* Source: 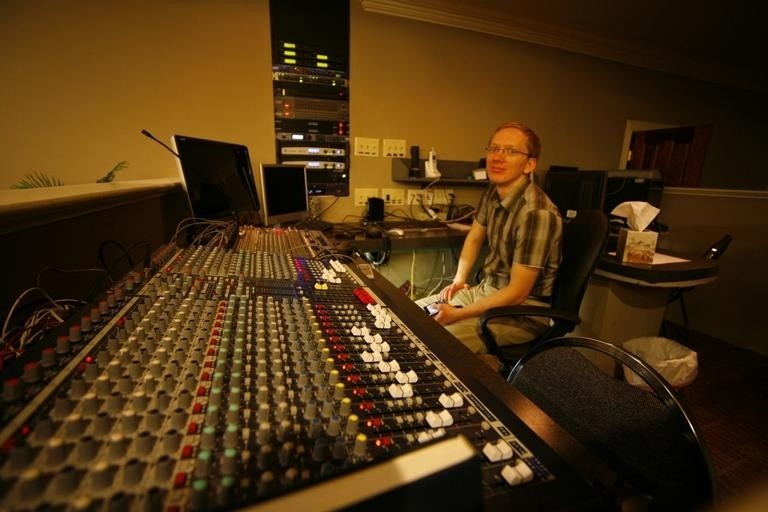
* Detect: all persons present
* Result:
[410,117,568,357]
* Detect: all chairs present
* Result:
[480,206,610,379]
[666,228,733,326]
[506,335,719,512]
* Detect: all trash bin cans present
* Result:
[622,336,699,394]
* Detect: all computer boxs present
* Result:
[604,177,664,219]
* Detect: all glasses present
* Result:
[485,145,535,158]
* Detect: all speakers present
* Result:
[366,197,384,222]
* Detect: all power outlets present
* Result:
[354,136,454,208]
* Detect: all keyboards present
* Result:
[310,221,332,231]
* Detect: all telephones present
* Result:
[425,147,442,178]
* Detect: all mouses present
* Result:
[334,230,356,239]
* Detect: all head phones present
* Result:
[363,228,391,267]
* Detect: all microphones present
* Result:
[140,128,180,158]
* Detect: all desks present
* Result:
[322,220,470,253]
[351,253,623,512]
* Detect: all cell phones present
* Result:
[423,300,444,316]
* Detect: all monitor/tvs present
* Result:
[545,169,605,218]
[259,163,310,227]
[170,133,261,222]
